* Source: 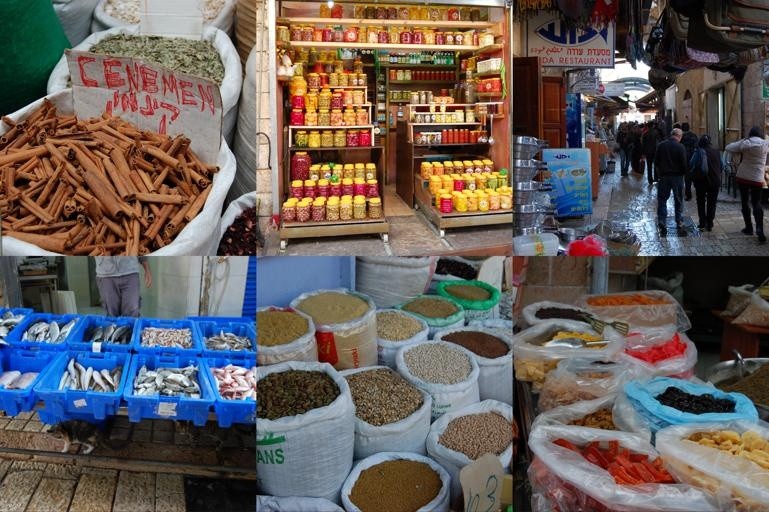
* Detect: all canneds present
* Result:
[276,1,513,224]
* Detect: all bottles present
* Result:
[472,32,478,46]
[412,70,455,81]
[397,102,404,120]
[378,49,454,65]
[442,128,468,143]
[350,48,374,58]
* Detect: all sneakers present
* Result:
[677,220,684,228]
[658,222,667,229]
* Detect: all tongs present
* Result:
[585,314,630,335]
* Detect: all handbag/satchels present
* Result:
[723,137,746,166]
[688,144,709,178]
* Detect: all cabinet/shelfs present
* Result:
[386,65,458,180]
[477,43,506,120]
[276,39,294,81]
[375,60,389,182]
[395,103,511,237]
[278,146,389,250]
[285,52,375,147]
[277,16,492,52]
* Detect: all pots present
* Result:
[558,227,587,242]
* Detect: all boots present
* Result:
[741,207,753,235]
[753,210,766,243]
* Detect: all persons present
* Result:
[726,126,767,243]
[690,134,723,229]
[655,128,689,230]
[94,256,151,316]
[616,120,698,199]
